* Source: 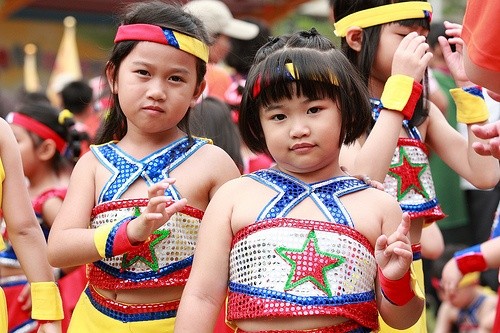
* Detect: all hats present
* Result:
[180,0,260,40]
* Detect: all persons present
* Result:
[0,76,116,333]
[46,0,241,333]
[331,0,500,333]
[181,0,259,176]
[172,26,424,333]
[0,116,65,333]
